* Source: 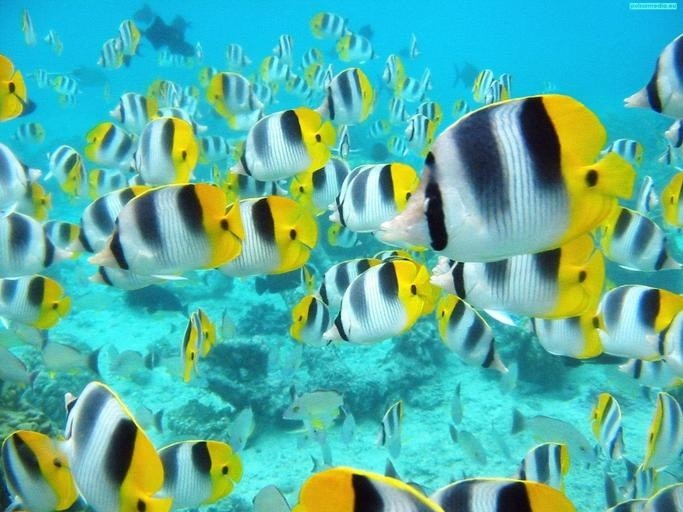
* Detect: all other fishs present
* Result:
[0,2,682,512]
